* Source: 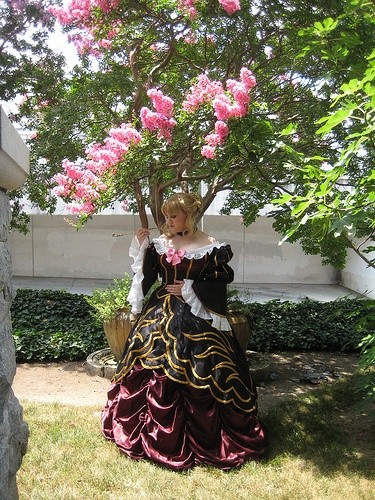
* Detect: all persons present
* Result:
[100,191,271,474]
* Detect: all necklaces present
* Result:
[175,227,196,236]
